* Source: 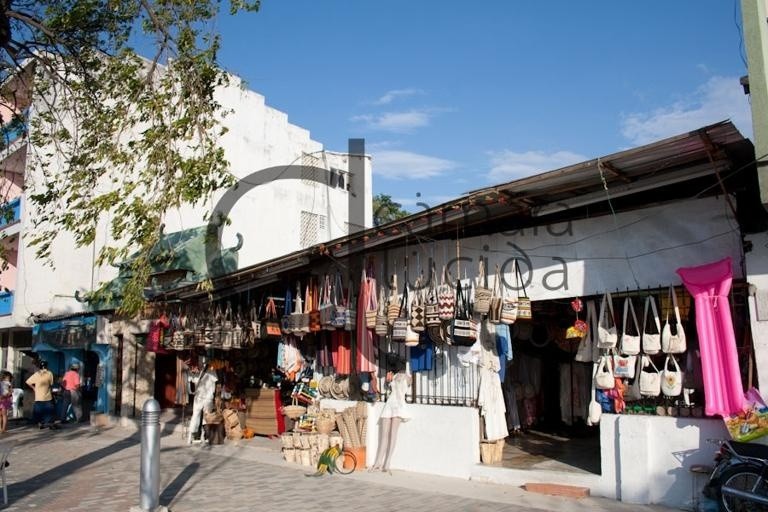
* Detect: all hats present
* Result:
[71,363,81,369]
[317,374,364,401]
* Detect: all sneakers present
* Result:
[39,423,62,431]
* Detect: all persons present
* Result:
[11,386,25,418]
[0,370,13,400]
[187,369,219,446]
[0,369,13,434]
[25,361,62,430]
[61,363,84,424]
[370,368,410,474]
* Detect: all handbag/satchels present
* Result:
[574,290,688,426]
[721,387,768,442]
[159,285,356,349]
[366,260,531,347]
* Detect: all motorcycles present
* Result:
[702,438,767,510]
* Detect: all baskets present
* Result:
[280,398,344,472]
